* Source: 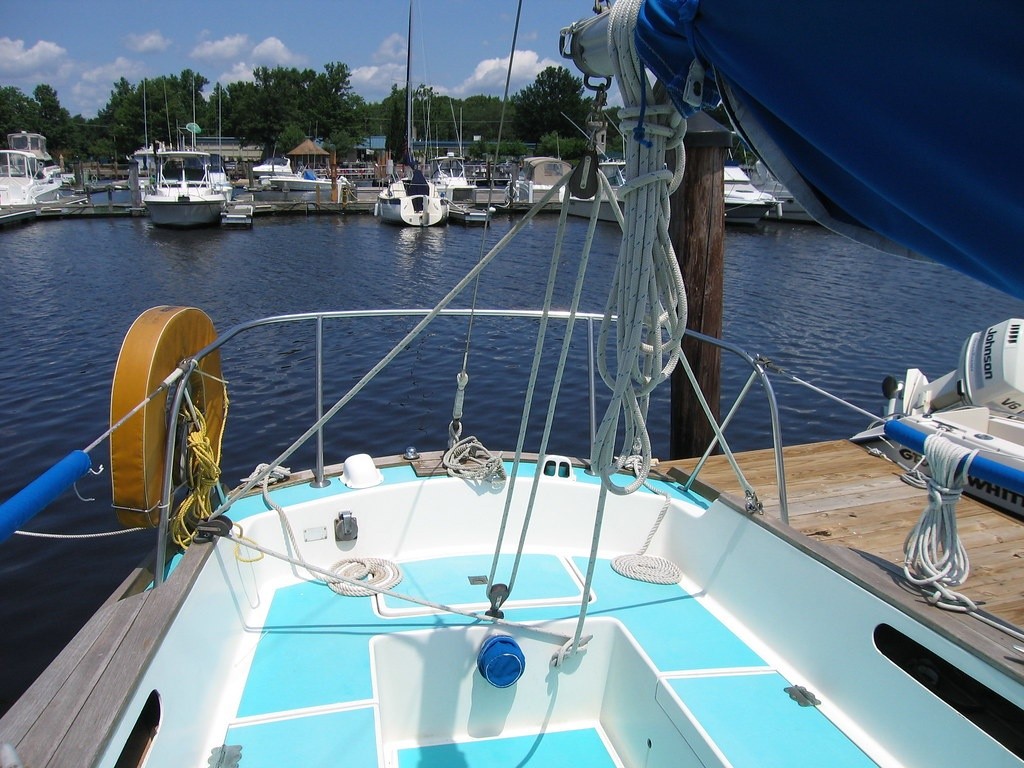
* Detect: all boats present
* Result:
[563,161,626,222]
[249,173,357,202]
[253,156,294,177]
[0,128,65,226]
[724,161,824,225]
[414,88,477,200]
[132,76,235,226]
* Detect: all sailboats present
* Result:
[375,0,446,228]
